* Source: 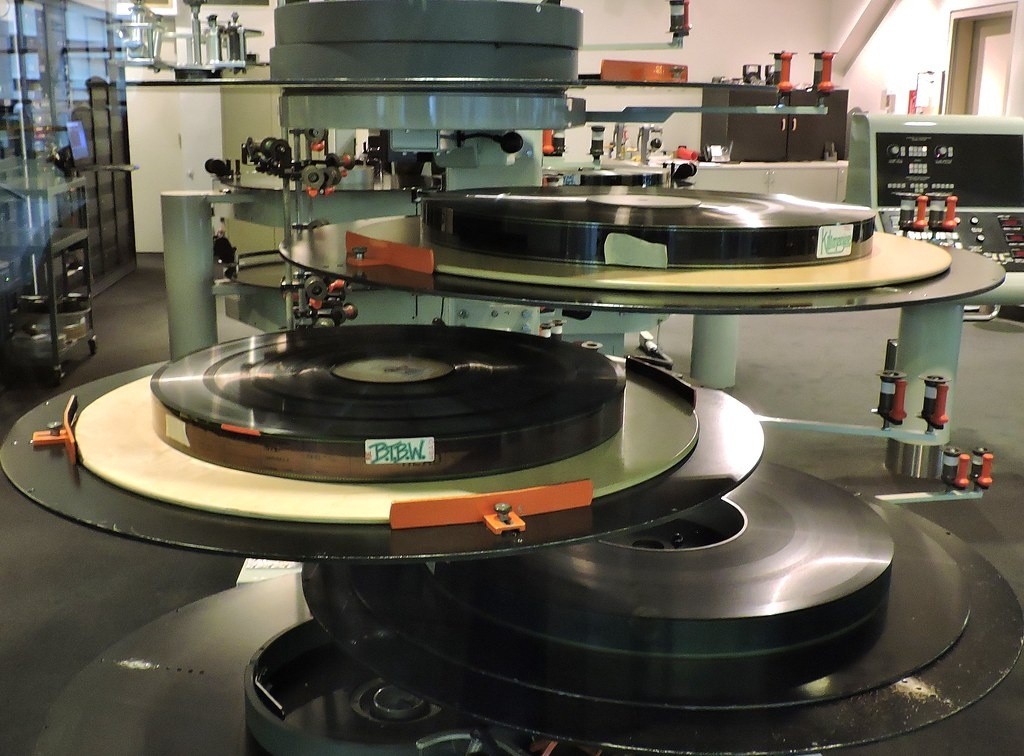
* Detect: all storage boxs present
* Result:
[600,59,688,83]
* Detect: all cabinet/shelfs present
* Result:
[0,175,98,385]
[700,86,849,163]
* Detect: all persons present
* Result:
[45,77,128,177]
